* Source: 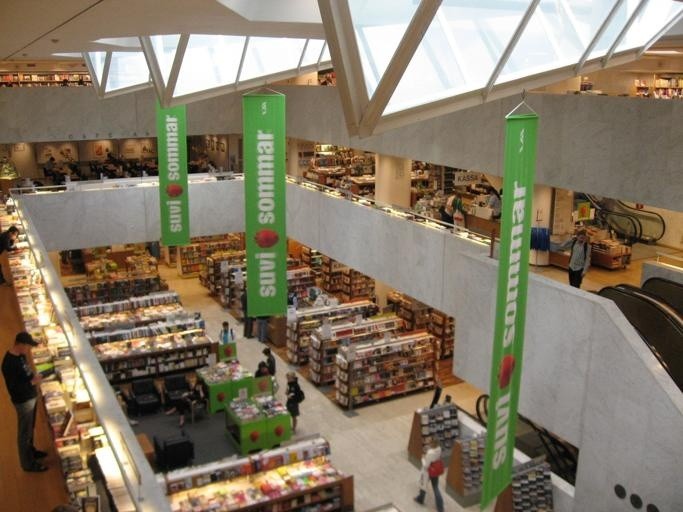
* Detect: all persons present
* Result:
[443,192,464,224]
[558,227,592,289]
[255,361,273,380]
[261,346,277,376]
[1,331,52,474]
[164,380,204,428]
[239,284,255,339]
[312,153,322,169]
[484,185,502,220]
[411,435,445,511]
[253,315,270,343]
[283,370,300,431]
[33,147,213,186]
[217,319,237,347]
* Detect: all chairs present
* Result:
[181,389,211,423]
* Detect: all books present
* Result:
[0,198,58,328]
[28,325,136,511]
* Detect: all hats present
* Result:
[16,331,39,346]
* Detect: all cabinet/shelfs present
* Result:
[169,454,354,511]
[406,403,555,511]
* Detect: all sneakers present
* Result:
[23,462,48,472]
[33,450,48,459]
